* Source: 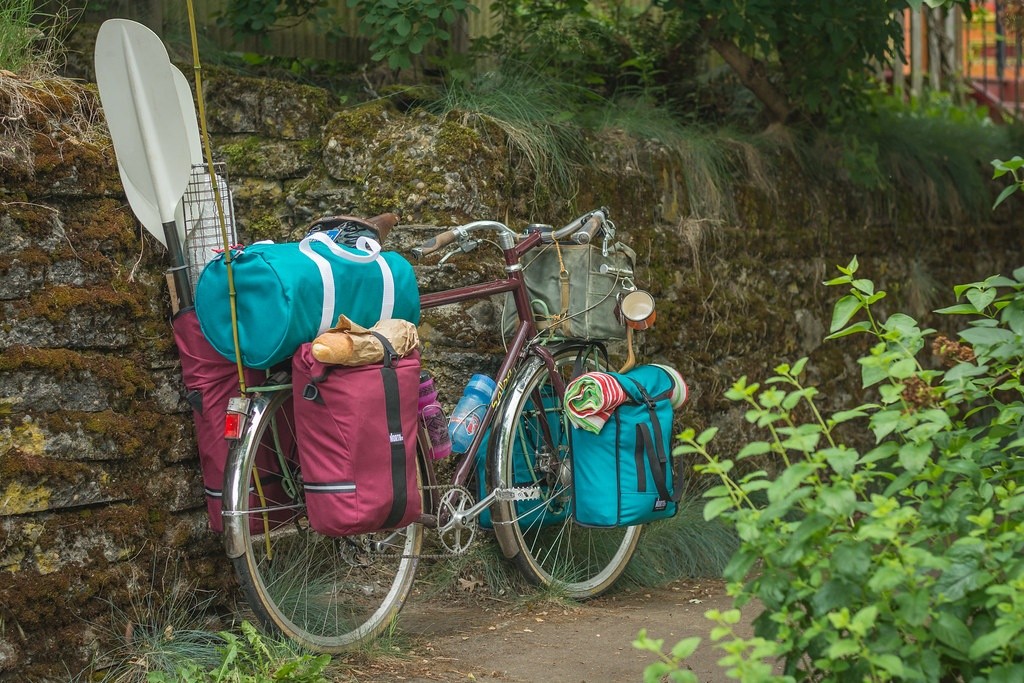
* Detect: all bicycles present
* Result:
[220,207,642,655]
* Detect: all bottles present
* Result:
[447,356,499,452]
[416,370,451,458]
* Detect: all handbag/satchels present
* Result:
[503,238,638,343]
[292,342,422,537]
[170,305,306,536]
[469,380,570,532]
[195,231,421,371]
[566,362,689,530]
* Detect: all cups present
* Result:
[621,288,656,331]
